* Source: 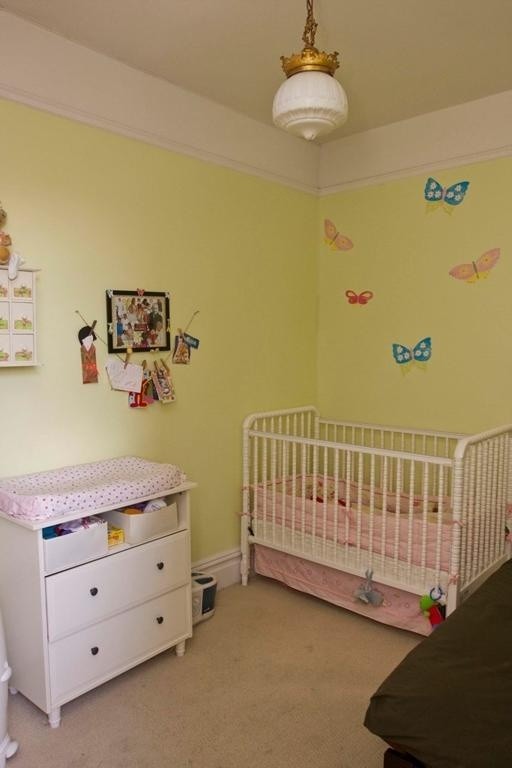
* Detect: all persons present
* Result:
[116,297,163,347]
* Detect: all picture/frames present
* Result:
[106,289,171,354]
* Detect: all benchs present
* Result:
[363,554,512,768]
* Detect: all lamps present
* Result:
[272,0,349,142]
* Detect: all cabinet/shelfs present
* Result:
[0,480,197,729]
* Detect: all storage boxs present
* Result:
[44,517,108,573]
[104,502,179,547]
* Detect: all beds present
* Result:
[239,405,512,638]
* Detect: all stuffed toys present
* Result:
[353,582,390,607]
[420,593,444,628]
